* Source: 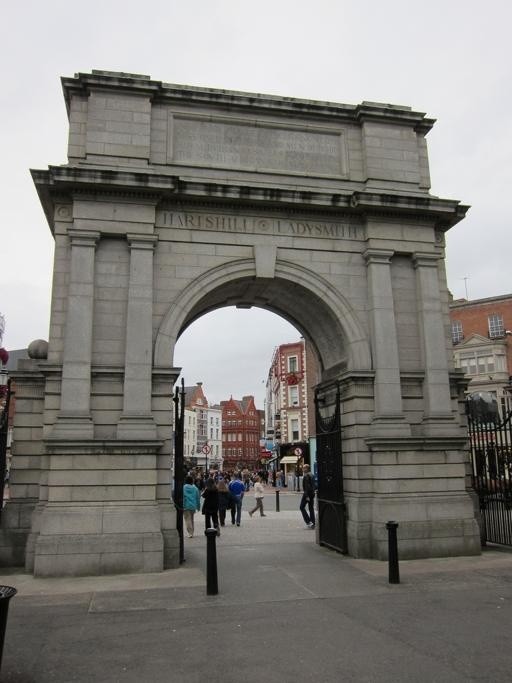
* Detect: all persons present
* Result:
[183,476,200,538]
[194,469,285,536]
[299,463,315,530]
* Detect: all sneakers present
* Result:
[304,522,314,529]
[187,535,192,538]
[261,514,265,516]
[232,521,240,526]
[249,512,252,517]
